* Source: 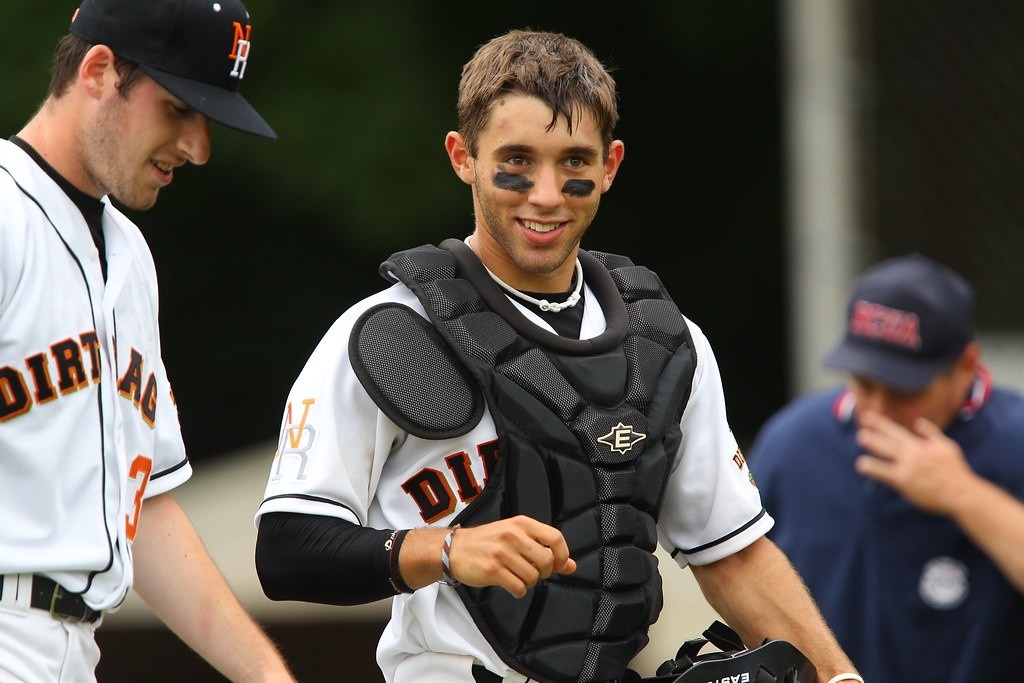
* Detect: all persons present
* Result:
[0,0,294,683]
[251,29,866,683]
[745,254,1024,683]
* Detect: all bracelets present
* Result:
[435,520,465,590]
[827,673,864,683]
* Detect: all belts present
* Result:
[0,574,103,626]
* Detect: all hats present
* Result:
[69,0,279,141]
[817,253,978,397]
